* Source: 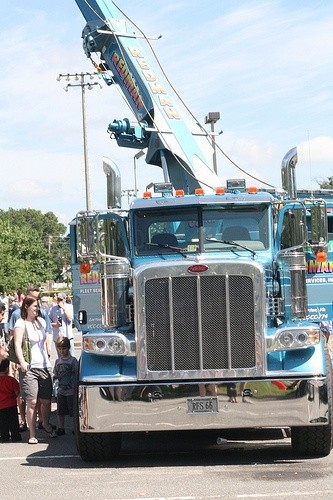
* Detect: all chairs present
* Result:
[150,233,178,248]
[221,226,250,240]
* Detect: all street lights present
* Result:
[57,72,103,211]
[134,151,145,199]
[121,189,139,208]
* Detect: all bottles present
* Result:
[52,377,59,397]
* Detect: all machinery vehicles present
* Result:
[56,1,332,464]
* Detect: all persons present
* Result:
[48,337,79,439]
[0,287,74,431]
[108,382,249,403]
[0,358,22,443]
[12,294,58,444]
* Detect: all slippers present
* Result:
[28,436,38,444]
[41,423,58,438]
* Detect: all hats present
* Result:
[27,285,39,293]
[57,293,66,299]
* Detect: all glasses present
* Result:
[57,298,63,301]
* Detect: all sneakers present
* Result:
[56,428,65,435]
[19,422,27,432]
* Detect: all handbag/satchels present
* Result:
[7,321,31,365]
